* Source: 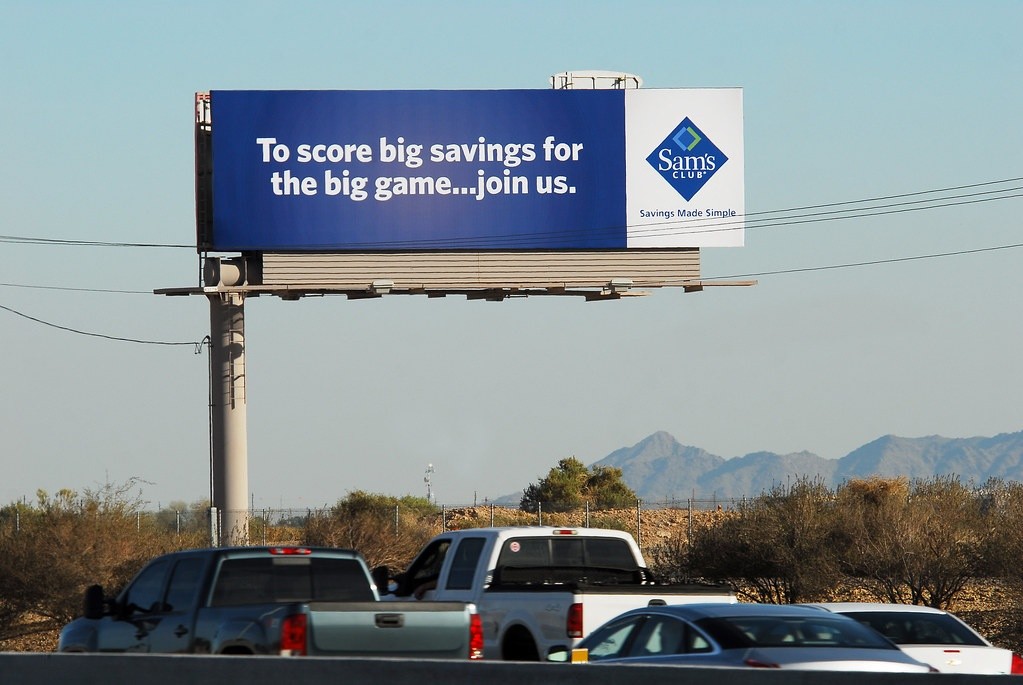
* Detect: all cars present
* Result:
[544,601,937,674]
[753,602,1023,677]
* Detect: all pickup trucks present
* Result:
[371,525,740,662]
[58,545,484,660]
